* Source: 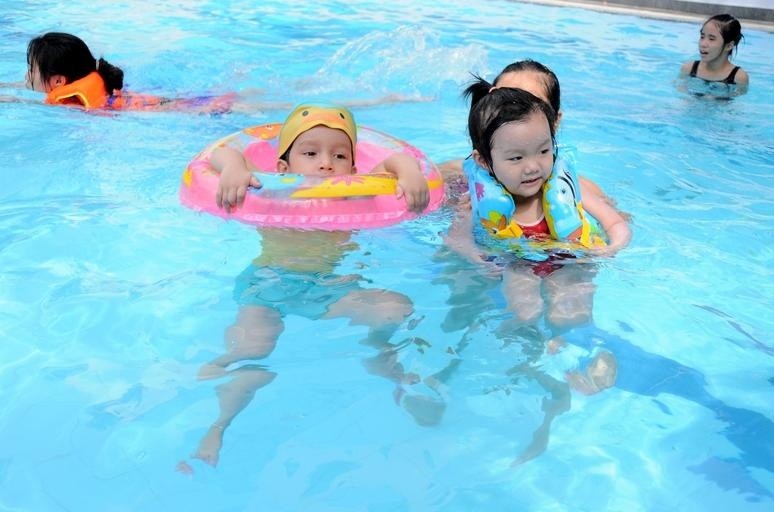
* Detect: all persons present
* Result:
[173,102,450,477]
[431,59,563,179]
[672,11,749,107]
[0,31,435,119]
[441,69,633,397]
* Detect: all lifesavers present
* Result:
[179,122,445,231]
[460,148,607,266]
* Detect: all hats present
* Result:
[277,101,357,165]
